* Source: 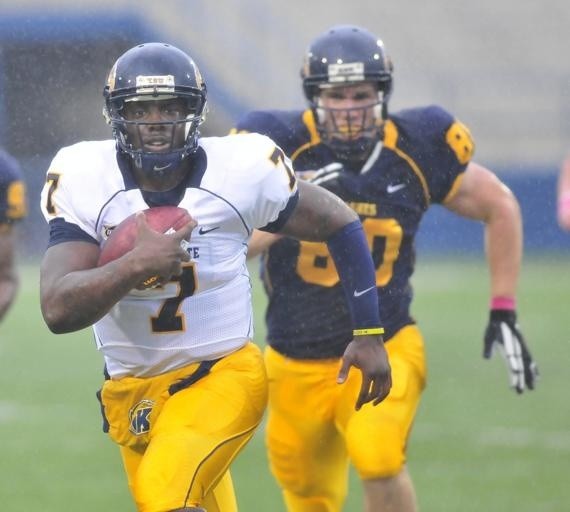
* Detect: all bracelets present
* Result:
[323,219,385,336]
[490,297,515,310]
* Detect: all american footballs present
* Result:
[97,207,191,294]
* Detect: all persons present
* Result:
[556,154,570,231]
[225,23,540,512]
[0,148,28,322]
[34,40,392,512]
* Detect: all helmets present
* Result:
[299,25,392,159]
[102,42,209,177]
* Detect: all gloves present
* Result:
[483,296,540,393]
